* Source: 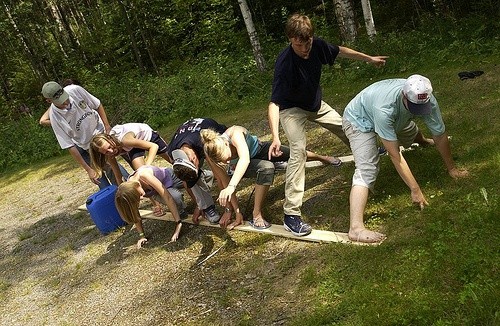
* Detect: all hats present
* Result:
[42,81,69,105]
[403,74,433,115]
[171,149,198,177]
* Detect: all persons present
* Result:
[87,120,183,197]
[115,167,216,251]
[200,125,342,230]
[39,78,131,191]
[340,75,469,243]
[268,14,389,236]
[167,111,289,224]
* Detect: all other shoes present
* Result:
[178,209,188,220]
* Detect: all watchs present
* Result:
[225,207,233,213]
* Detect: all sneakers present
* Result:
[377,146,387,156]
[203,204,221,225]
[284,214,312,236]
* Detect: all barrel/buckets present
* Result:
[85,185,128,237]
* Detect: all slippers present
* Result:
[322,156,341,166]
[249,219,272,229]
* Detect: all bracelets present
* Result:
[233,208,241,214]
[175,219,182,225]
[138,232,145,236]
